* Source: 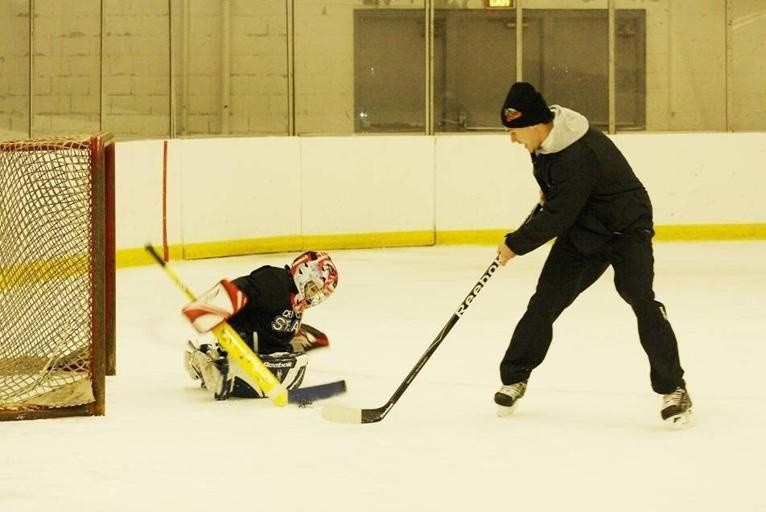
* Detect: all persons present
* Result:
[493,82,693,420]
[182,247,341,400]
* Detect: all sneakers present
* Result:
[494,379,528,406]
[660,377,692,420]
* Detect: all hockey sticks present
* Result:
[145,244,346,405]
[321,199,547,423]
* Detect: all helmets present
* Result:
[290,251,338,314]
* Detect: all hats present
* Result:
[501,82,554,128]
